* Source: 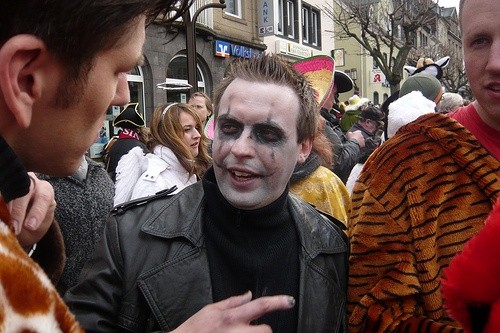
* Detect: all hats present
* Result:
[344,95,369,112]
[333,69,355,103]
[398,73,443,104]
[113,103,145,128]
[287,54,335,114]
[360,104,385,125]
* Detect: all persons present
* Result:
[0,0,500,333]
